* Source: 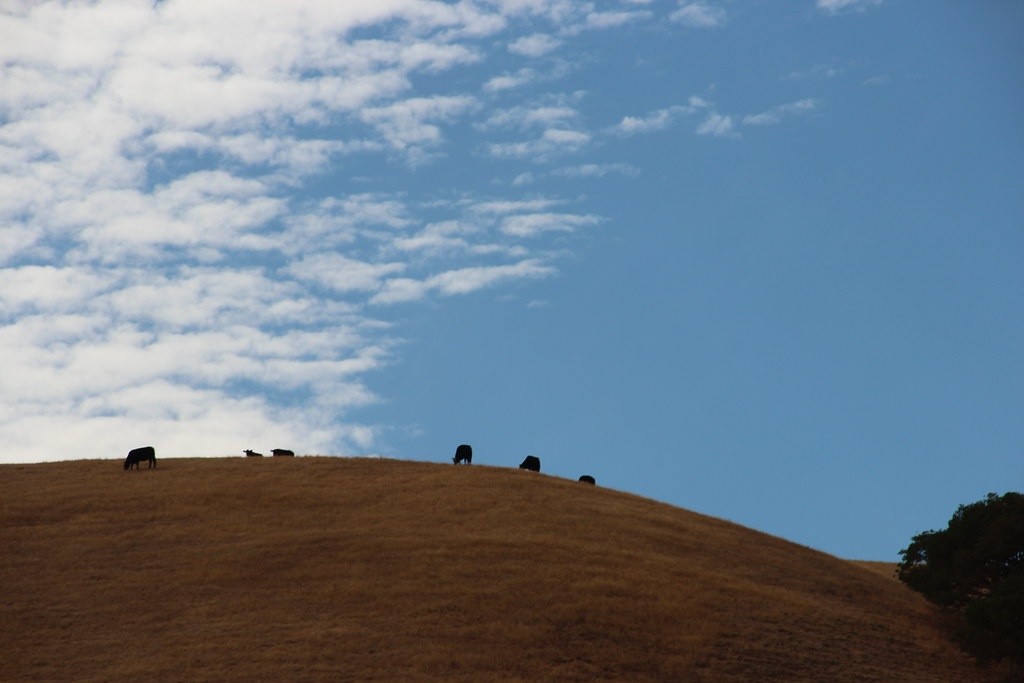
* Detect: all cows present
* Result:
[578,476,595,484]
[243,450,262,457]
[519,456,540,472]
[271,449,294,456]
[124,447,157,470]
[452,445,472,465]
